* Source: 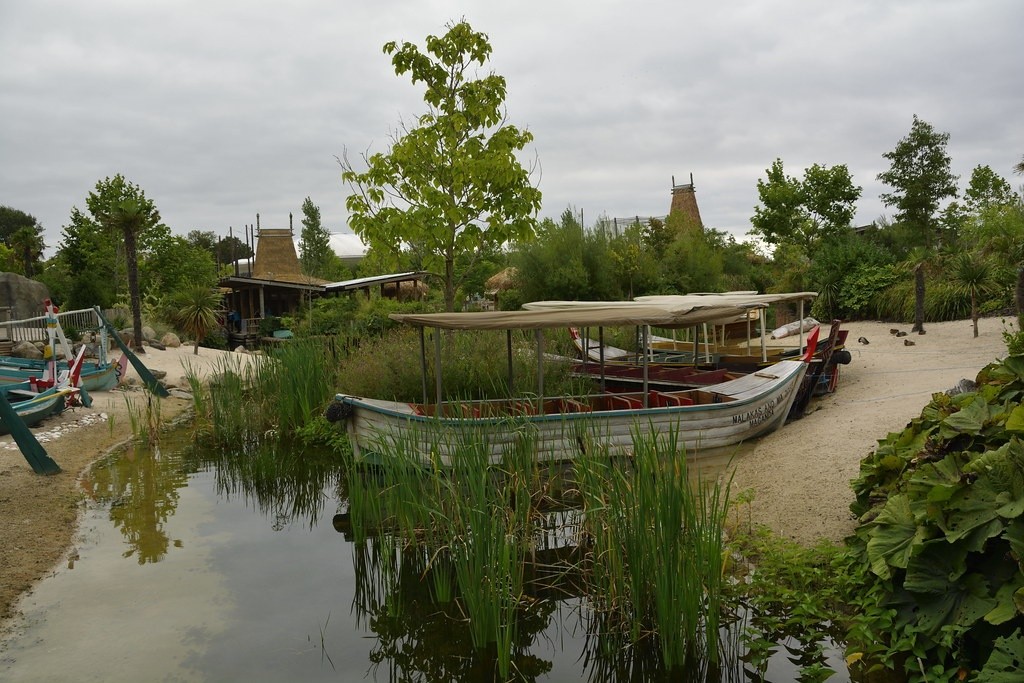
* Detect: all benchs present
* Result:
[556,398,591,412]
[576,353,728,387]
[461,403,480,418]
[9,388,41,397]
[604,390,643,411]
[507,400,536,416]
[648,389,693,408]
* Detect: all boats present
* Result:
[323,289,851,482]
[0,337,132,434]
